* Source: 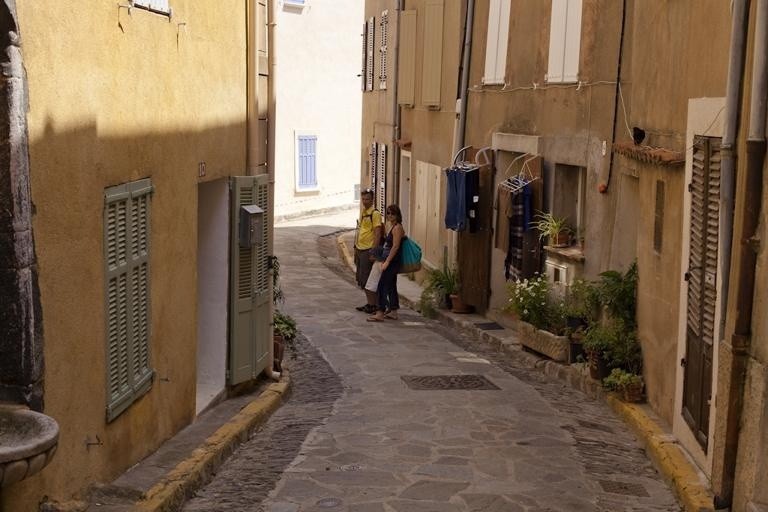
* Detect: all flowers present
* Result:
[513,269,586,333]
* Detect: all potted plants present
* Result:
[578,327,616,381]
[269,303,300,369]
[602,369,645,404]
[425,265,473,314]
[534,212,573,248]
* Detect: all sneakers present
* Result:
[356,304,377,313]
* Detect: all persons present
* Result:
[354,189,391,314]
[367,205,405,321]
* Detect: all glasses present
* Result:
[387,212,396,215]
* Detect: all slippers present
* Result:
[368,316,384,322]
[383,314,397,319]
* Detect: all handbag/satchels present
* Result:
[398,237,421,273]
